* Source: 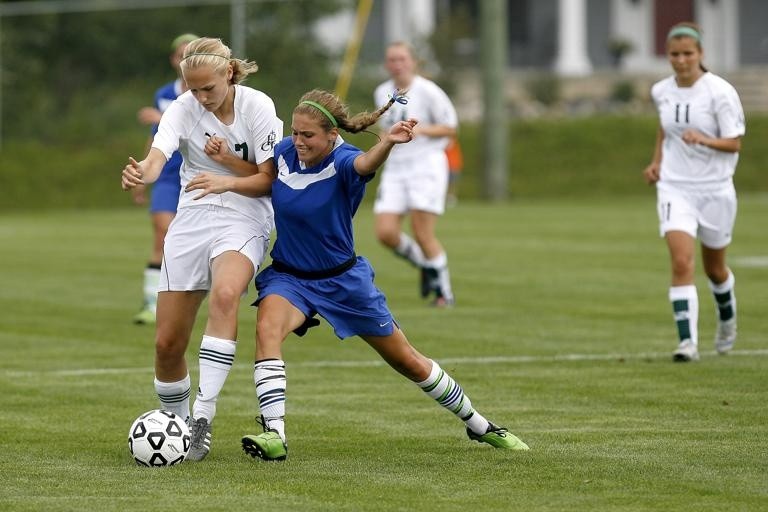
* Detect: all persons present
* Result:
[203,88,531,461]
[120,37,285,461]
[133,33,201,326]
[642,20,746,361]
[372,43,459,306]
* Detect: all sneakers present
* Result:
[420,267,432,298]
[242,429,288,461]
[467,421,528,450]
[187,417,212,461]
[713,318,737,355]
[130,302,157,326]
[429,295,456,307]
[671,337,702,362]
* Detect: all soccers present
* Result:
[128,408,190,465]
